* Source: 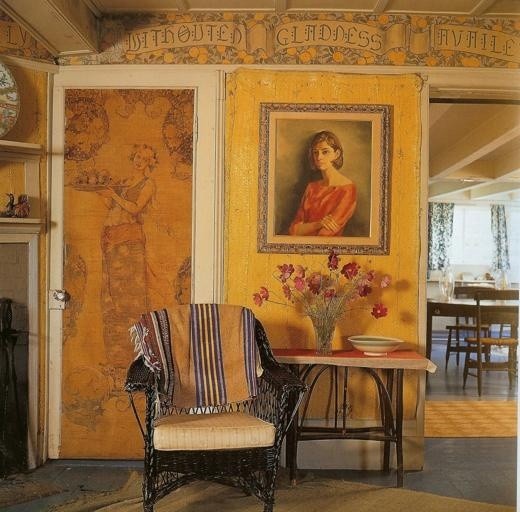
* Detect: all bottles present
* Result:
[495,268,511,290]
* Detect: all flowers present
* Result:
[251,253,391,354]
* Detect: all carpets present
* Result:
[424,398,518,439]
[1,464,515,512]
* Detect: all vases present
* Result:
[310,315,338,356]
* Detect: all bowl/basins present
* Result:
[348,335,405,357]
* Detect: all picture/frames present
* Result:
[255,100,394,254]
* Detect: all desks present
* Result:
[426,299,518,374]
[269,348,427,488]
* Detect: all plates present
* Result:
[0,62,22,140]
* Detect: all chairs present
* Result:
[446,286,519,397]
[122,302,309,512]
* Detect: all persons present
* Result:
[287,129,359,236]
[94,142,160,381]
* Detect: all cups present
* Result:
[438,271,455,301]
